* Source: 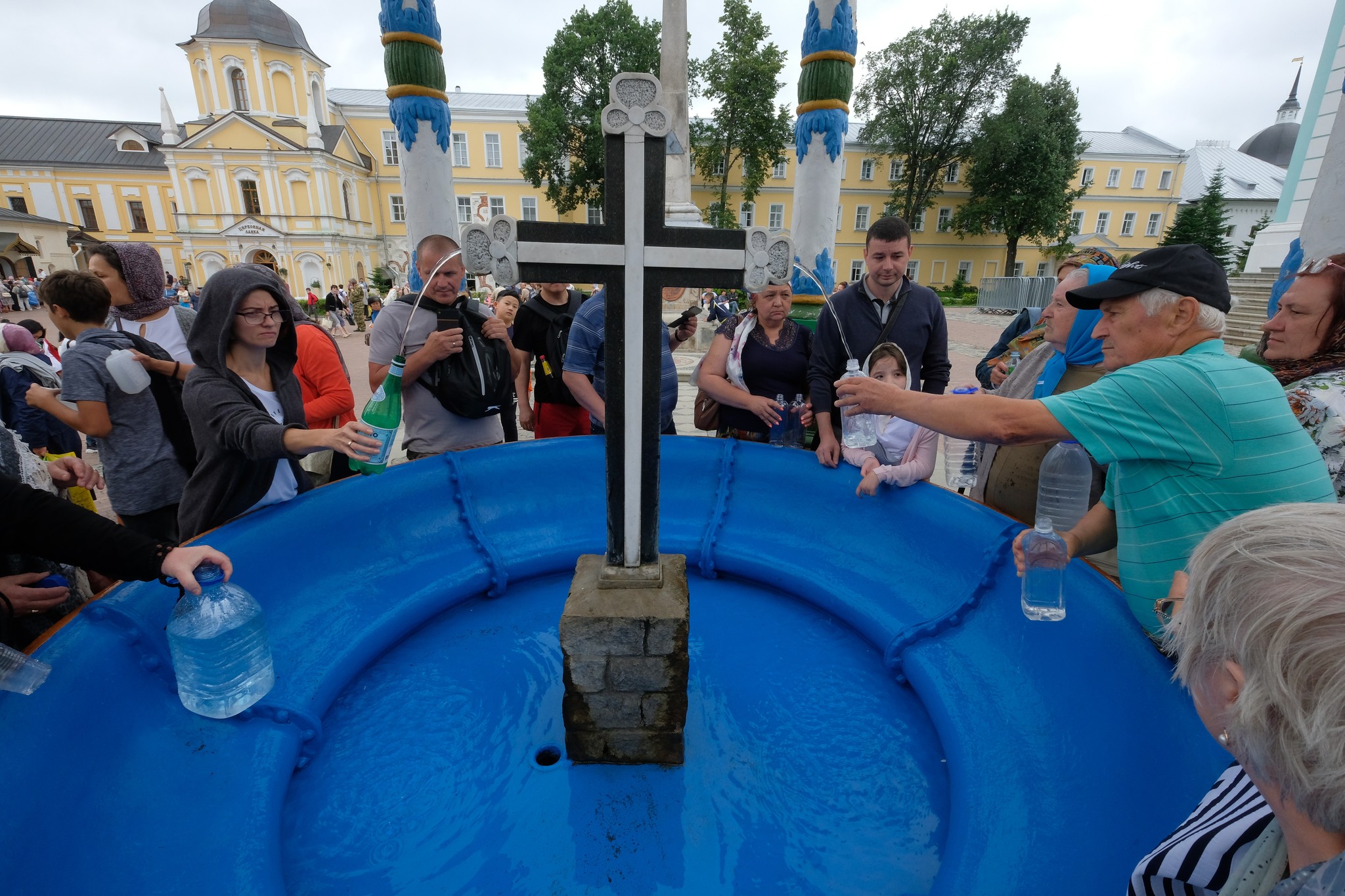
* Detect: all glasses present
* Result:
[371,303,381,307]
[1153,595,1193,638]
[488,293,491,296]
[1294,253,1344,280]
[234,309,291,326]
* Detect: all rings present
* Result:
[348,441,355,448]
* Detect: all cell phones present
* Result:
[681,305,703,317]
[436,309,459,332]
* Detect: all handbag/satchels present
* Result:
[367,288,370,293]
[162,296,167,301]
[694,312,743,434]
[2,292,12,298]
[288,443,334,489]
[345,314,356,326]
[321,315,333,330]
[183,298,190,305]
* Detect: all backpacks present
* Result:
[338,290,345,298]
[522,289,590,413]
[391,293,516,422]
[16,286,29,300]
[522,286,537,303]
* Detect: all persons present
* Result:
[806,216,953,470]
[0,222,233,676]
[305,276,594,441]
[228,263,360,488]
[512,280,599,440]
[955,244,1120,497]
[969,264,1128,589]
[842,342,940,498]
[836,281,850,293]
[560,282,703,435]
[833,241,1336,652]
[698,287,744,329]
[366,235,523,460]
[697,277,818,451]
[177,264,382,541]
[1260,254,1345,505]
[1127,504,1345,896]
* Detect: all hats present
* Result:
[1065,242,1230,314]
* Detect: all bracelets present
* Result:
[674,329,688,342]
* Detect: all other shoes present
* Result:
[333,332,341,336]
[332,336,335,338]
[343,335,349,339]
[346,333,352,335]
[353,319,372,332]
[5,309,14,312]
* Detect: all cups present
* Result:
[167,566,274,719]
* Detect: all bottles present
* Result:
[1004,351,1021,375]
[1034,440,1092,532]
[769,394,789,447]
[840,359,877,448]
[1021,516,1068,622]
[786,394,808,449]
[349,356,406,476]
[944,387,980,487]
[105,350,152,394]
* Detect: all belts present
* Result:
[350,300,364,305]
